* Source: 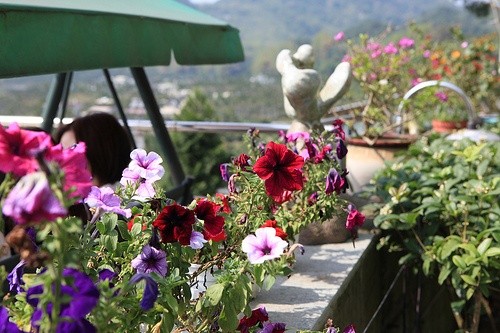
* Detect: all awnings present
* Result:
[1,0,250,207]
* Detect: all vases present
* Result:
[346,135,420,203]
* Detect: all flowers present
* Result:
[0,114,365,333]
[330,32,462,146]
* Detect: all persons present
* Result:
[55,110,135,187]
